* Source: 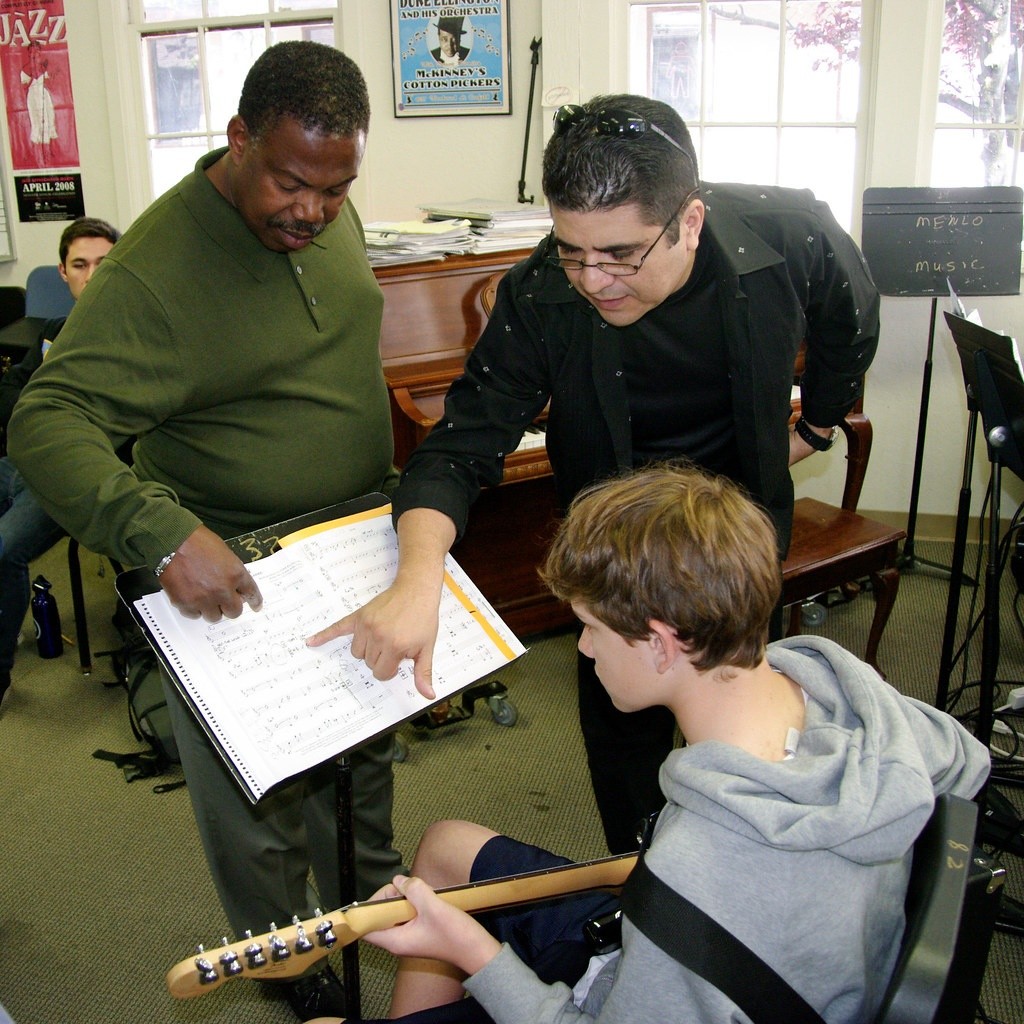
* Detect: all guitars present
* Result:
[166,835,642,1004]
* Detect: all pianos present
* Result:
[373,243,875,644]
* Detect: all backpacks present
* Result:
[123,643,184,765]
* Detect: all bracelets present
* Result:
[154,553,175,576]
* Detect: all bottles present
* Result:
[31,573,64,659]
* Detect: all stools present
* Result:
[772,498,911,686]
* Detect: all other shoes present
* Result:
[0,673,11,710]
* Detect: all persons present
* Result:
[305,468,989,1024]
[8,40,410,1022]
[303,94,882,859]
[0,216,117,699]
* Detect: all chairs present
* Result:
[26,263,77,318]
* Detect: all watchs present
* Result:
[794,415,840,452]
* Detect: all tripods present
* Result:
[860,186,1024,593]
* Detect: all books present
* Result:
[361,198,554,269]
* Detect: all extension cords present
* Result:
[1007,686,1024,710]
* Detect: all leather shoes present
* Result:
[278,965,349,1022]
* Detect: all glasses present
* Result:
[541,182,701,277]
[551,103,700,190]
